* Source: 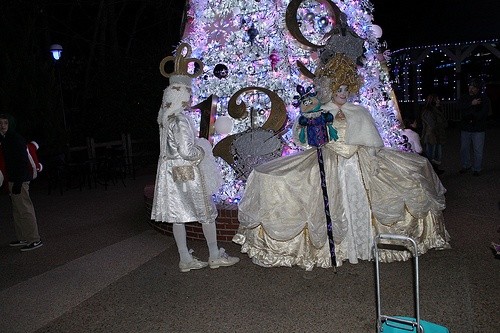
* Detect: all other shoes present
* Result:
[179,248,208,271]
[209,247,240,268]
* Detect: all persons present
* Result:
[232,51,450,271]
[150,43,240,272]
[0,113,43,252]
[420,94,448,175]
[458,79,489,176]
[403,114,423,153]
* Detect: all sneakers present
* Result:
[20,238,43,251]
[9,239,22,246]
